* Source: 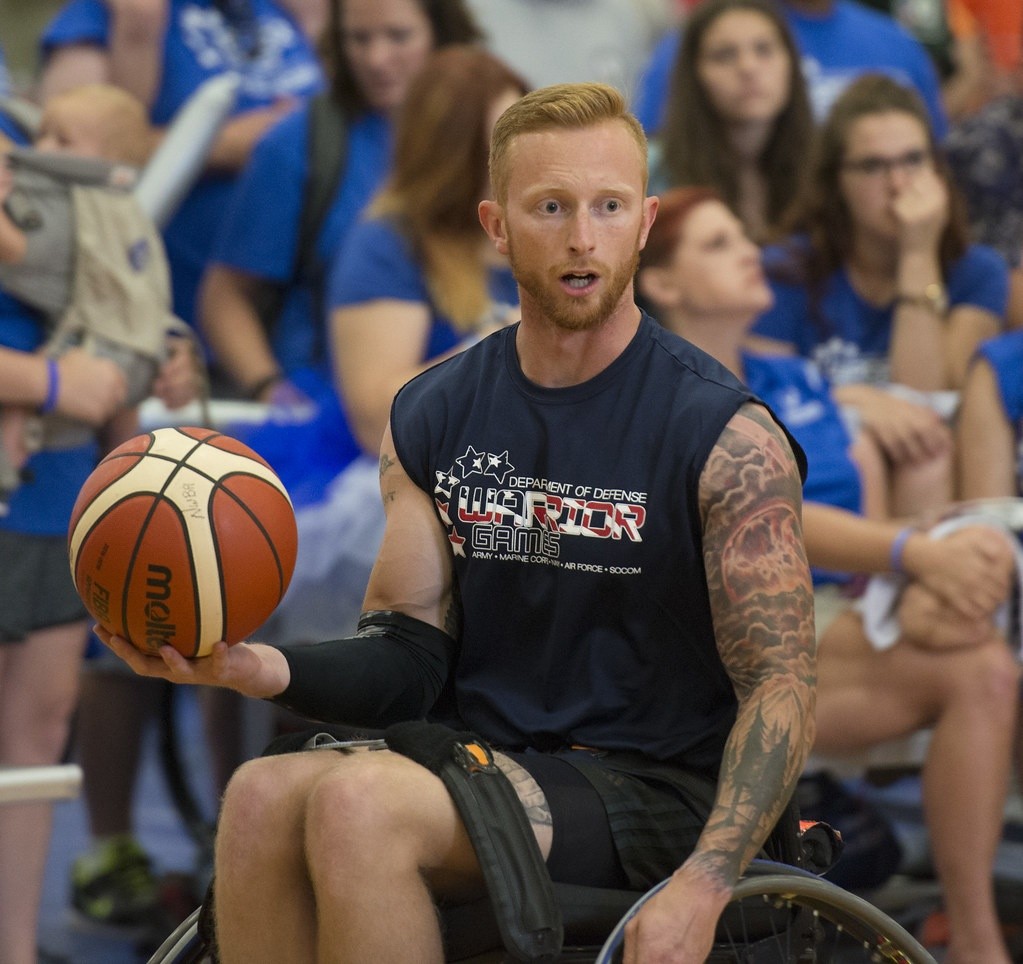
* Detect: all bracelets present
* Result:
[40,353,61,414]
[899,285,952,321]
[252,373,276,397]
[893,527,920,572]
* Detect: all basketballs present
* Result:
[67,426,299,658]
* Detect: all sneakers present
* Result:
[69,836,163,923]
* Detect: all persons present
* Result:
[0,0,1023,964]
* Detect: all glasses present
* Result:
[839,148,929,175]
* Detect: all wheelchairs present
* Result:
[142,781,934,964]
